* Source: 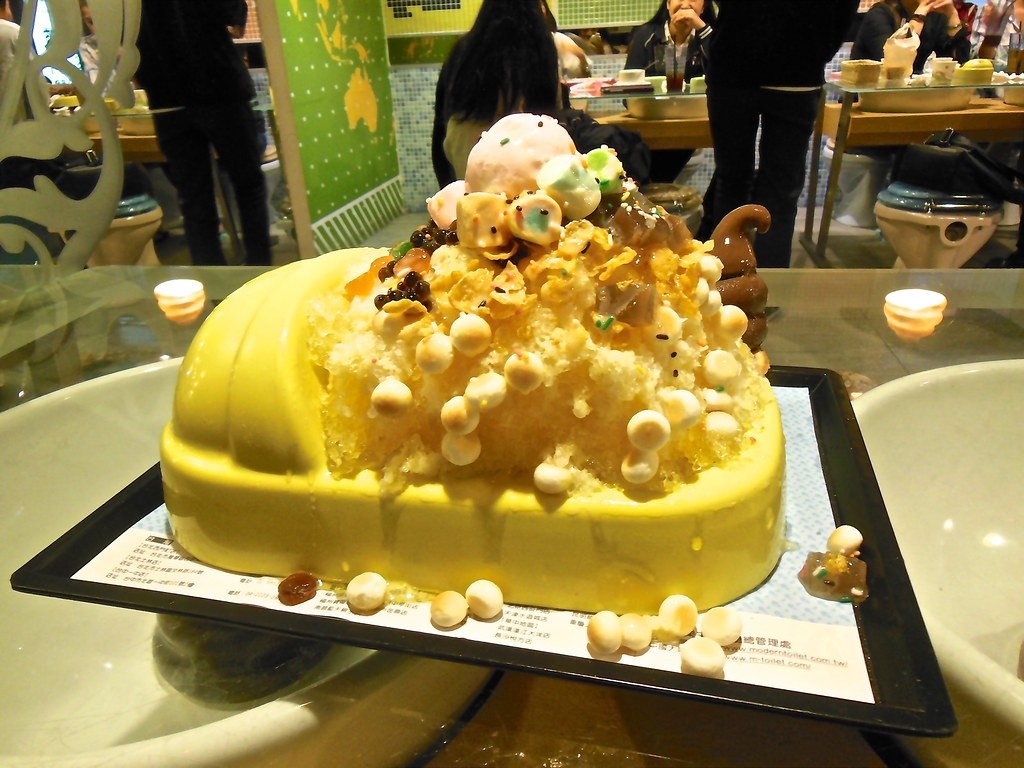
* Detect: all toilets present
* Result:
[822,136,893,228]
[872,179,1005,270]
[47,190,165,269]
[218,143,287,233]
[638,182,705,238]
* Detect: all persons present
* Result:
[121,0,271,266]
[624,0,716,184]
[839,0,972,153]
[432,0,563,190]
[78,0,169,243]
[570,28,619,54]
[0,0,94,202]
[693,0,861,269]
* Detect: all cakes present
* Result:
[154,111,785,618]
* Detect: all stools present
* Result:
[874,180,1004,269]
[51,191,163,265]
[640,182,704,238]
[823,136,896,228]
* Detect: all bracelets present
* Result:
[945,22,962,30]
[912,14,926,23]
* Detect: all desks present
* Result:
[63,112,241,256]
[0,262,1024,768]
[569,89,714,149]
[799,78,1024,269]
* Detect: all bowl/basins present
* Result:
[835,356,1024,766]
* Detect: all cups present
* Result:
[931,60,957,86]
[618,69,645,82]
